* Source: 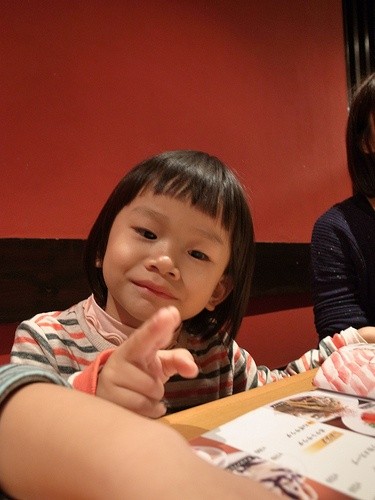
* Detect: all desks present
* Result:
[149,362,319,444]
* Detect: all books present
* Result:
[187,344,375,500]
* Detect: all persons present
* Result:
[0,364,287,500]
[11,150,375,418]
[311,74,375,350]
